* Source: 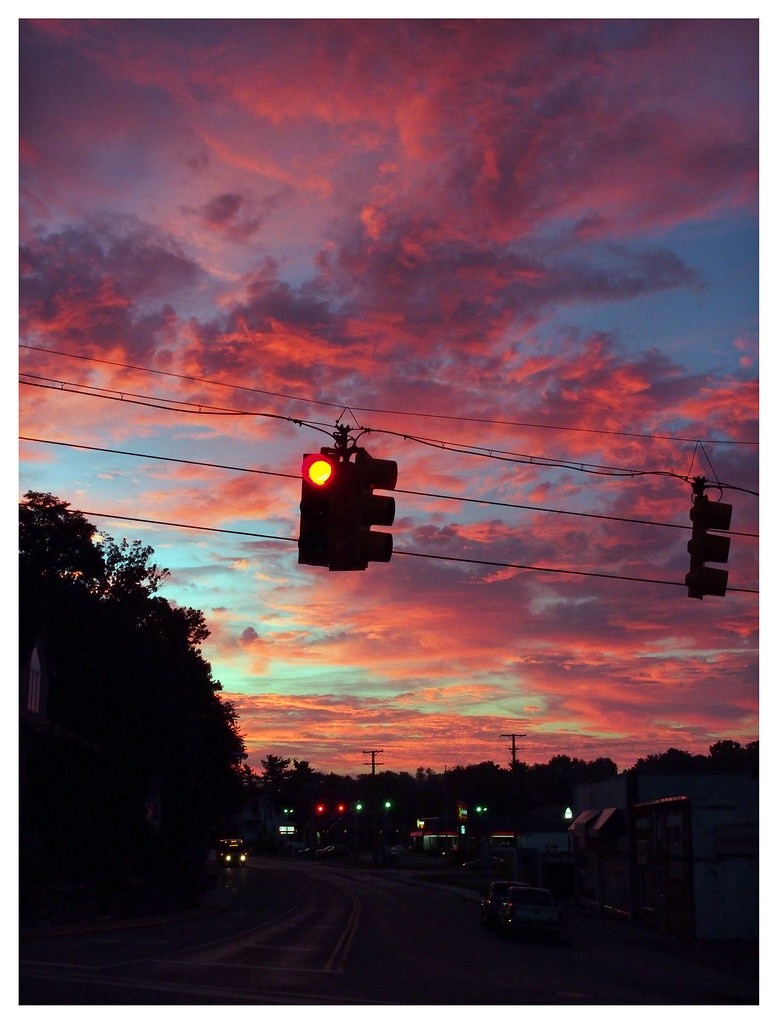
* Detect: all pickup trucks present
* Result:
[498,887,561,941]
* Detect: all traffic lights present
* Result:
[298,453,342,566]
[685,495,733,600]
[355,451,397,562]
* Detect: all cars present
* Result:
[297,843,345,860]
[216,838,249,867]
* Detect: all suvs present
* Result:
[480,881,531,932]
